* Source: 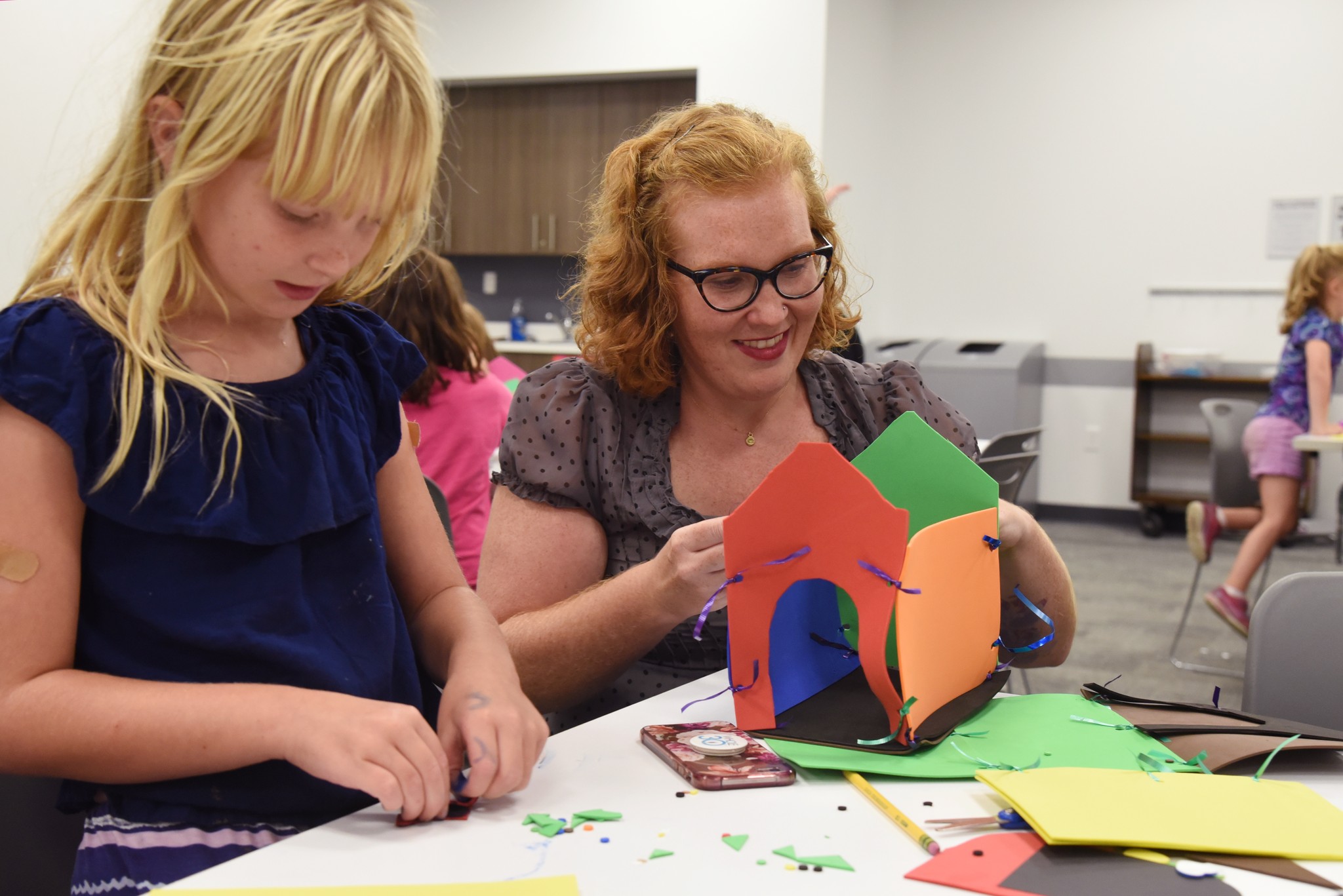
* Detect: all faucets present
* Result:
[544,308,574,340]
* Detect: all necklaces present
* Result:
[687,392,783,445]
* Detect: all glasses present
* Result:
[668,228,834,313]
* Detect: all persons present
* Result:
[473,100,1076,736]
[0,0,549,896]
[1185,244,1343,638]
[342,246,515,593]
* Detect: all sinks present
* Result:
[537,339,576,347]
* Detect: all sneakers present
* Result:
[1206,586,1250,636]
[1186,499,1217,564]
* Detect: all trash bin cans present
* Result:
[917,339,1045,519]
[858,336,940,378]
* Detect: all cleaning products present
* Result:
[510,298,527,341]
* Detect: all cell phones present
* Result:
[641,720,799,789]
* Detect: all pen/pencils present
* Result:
[840,769,942,852]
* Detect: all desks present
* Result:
[152,670,1343,896]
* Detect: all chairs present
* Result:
[978,427,1044,507]
[1239,571,1343,733]
[1166,400,1342,680]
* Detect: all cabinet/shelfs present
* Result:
[440,78,694,257]
[1125,342,1320,542]
